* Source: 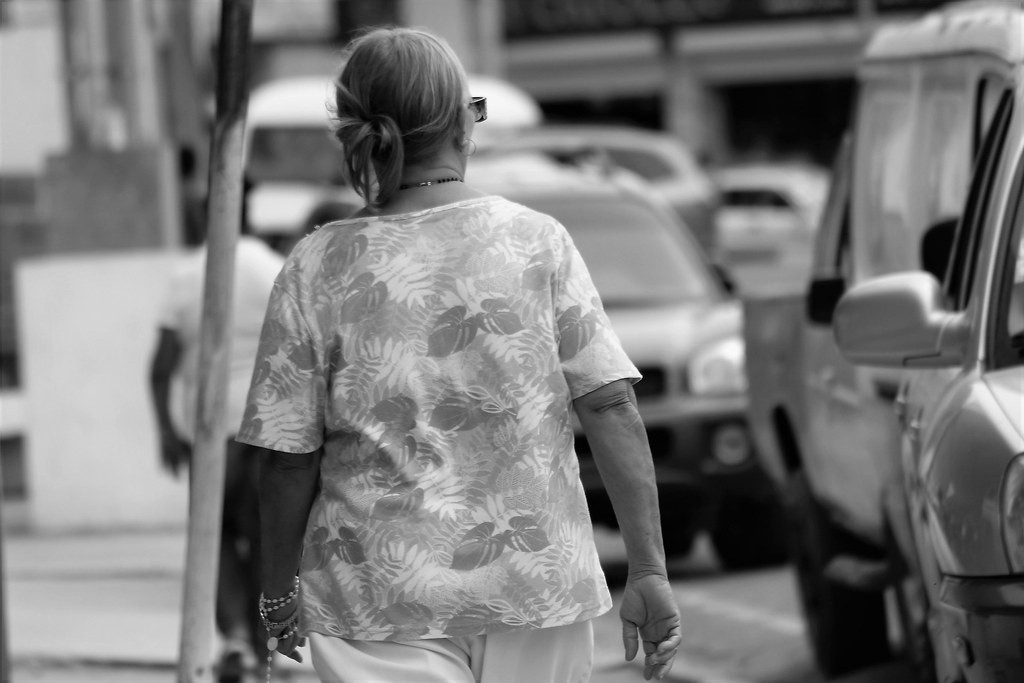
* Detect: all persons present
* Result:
[213,28,682,683]
[150,170,301,681]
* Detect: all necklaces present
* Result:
[395,177,464,189]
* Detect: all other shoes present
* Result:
[216,651,242,683]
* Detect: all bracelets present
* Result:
[258,576,299,683]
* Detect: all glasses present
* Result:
[467,96,488,123]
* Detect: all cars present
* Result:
[742,2,1024,683]
[220,70,827,562]
